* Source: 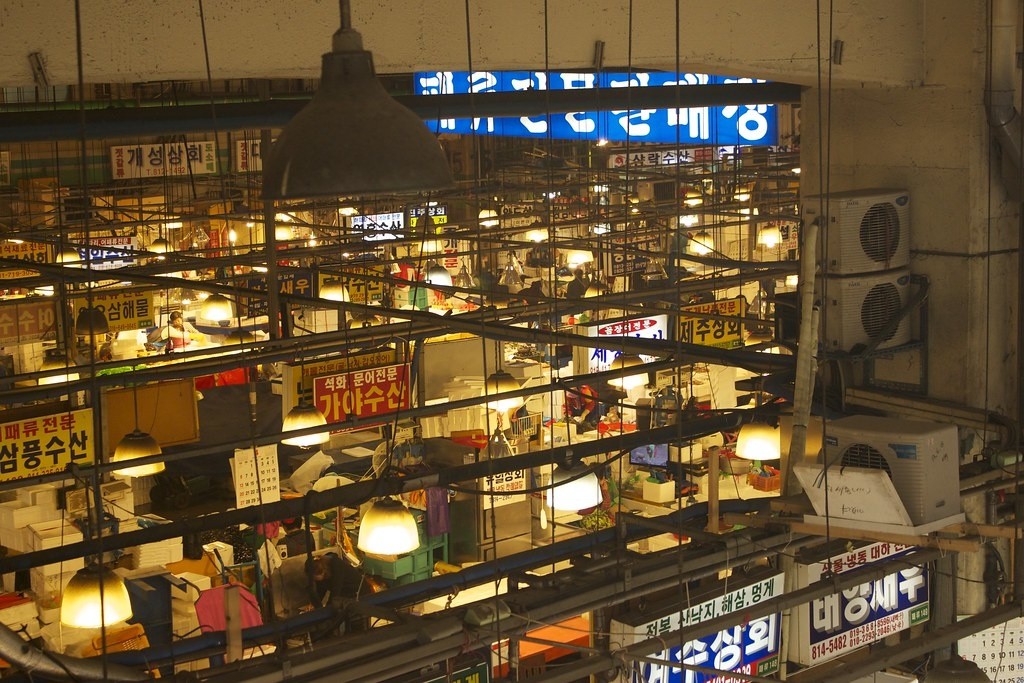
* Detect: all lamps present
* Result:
[197,292,232,322]
[60,479,133,629]
[55,248,80,267]
[151,224,170,254]
[110,367,166,477]
[734,424,780,461]
[258,0,456,201]
[37,354,79,387]
[320,210,783,408]
[743,331,779,354]
[924,547,994,683]
[356,420,418,556]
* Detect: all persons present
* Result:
[567,269,589,299]
[517,281,545,305]
[379,254,391,274]
[735,294,758,332]
[303,552,342,608]
[170,311,199,333]
[566,385,601,424]
[276,516,315,558]
[724,431,737,444]
[578,462,614,527]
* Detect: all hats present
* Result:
[566,387,579,398]
[170,311,182,321]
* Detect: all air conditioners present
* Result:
[822,271,912,352]
[824,414,960,526]
[547,390,604,511]
[804,188,911,276]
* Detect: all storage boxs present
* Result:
[853,672,919,683]
[1,361,777,677]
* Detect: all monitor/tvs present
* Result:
[629,444,669,469]
